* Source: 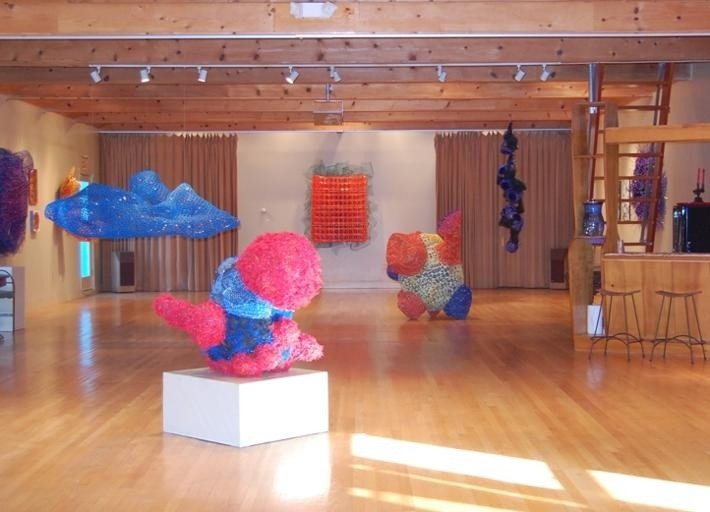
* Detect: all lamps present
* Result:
[290,2,337,18]
[88,62,562,84]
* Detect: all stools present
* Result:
[588,288,706,364]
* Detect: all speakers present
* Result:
[110,251,136,292]
[549,248,568,290]
